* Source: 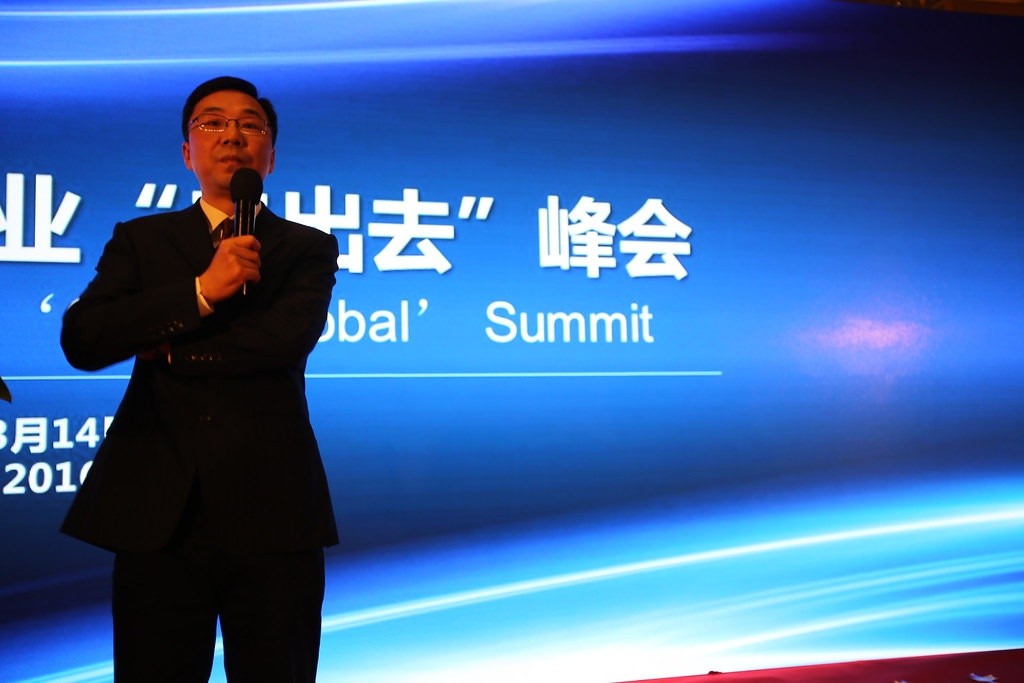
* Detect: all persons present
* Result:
[58,76,339,683]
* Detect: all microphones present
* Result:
[229,167,263,298]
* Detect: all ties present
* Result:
[215,218,234,253]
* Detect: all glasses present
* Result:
[186,112,271,136]
